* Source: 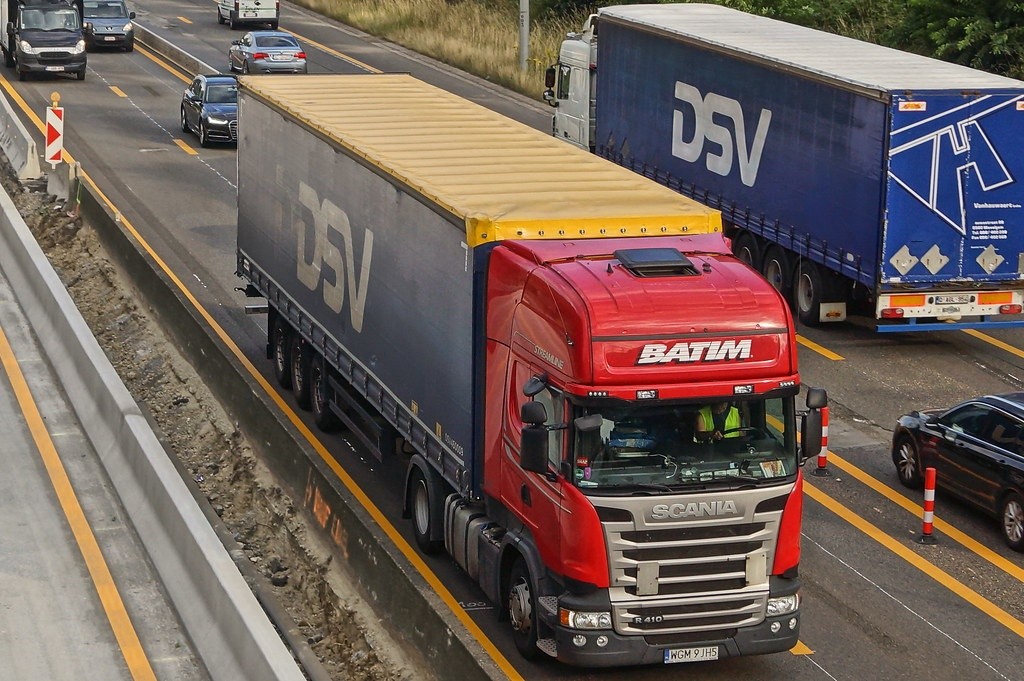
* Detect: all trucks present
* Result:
[1,0,94,80]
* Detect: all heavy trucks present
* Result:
[232,76,825,669]
[545,4,1024,332]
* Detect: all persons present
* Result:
[694,400,745,444]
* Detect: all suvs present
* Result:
[889,392,1024,554]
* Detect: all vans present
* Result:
[218,0,280,29]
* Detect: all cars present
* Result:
[78,0,145,50]
[229,31,309,75]
[179,73,241,147]
